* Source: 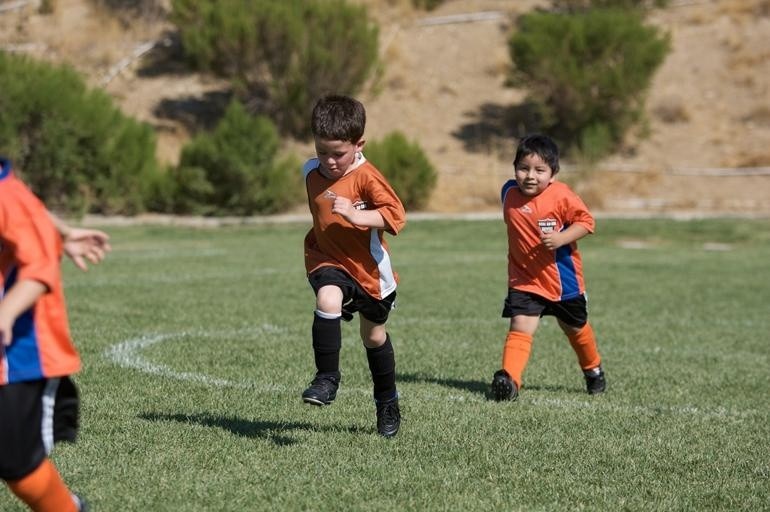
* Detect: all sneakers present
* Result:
[491,368,519,401]
[301,374,340,407]
[584,363,605,394]
[374,391,401,438]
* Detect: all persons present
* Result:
[1,153,111,511]
[298,92,408,435]
[489,133,607,400]
